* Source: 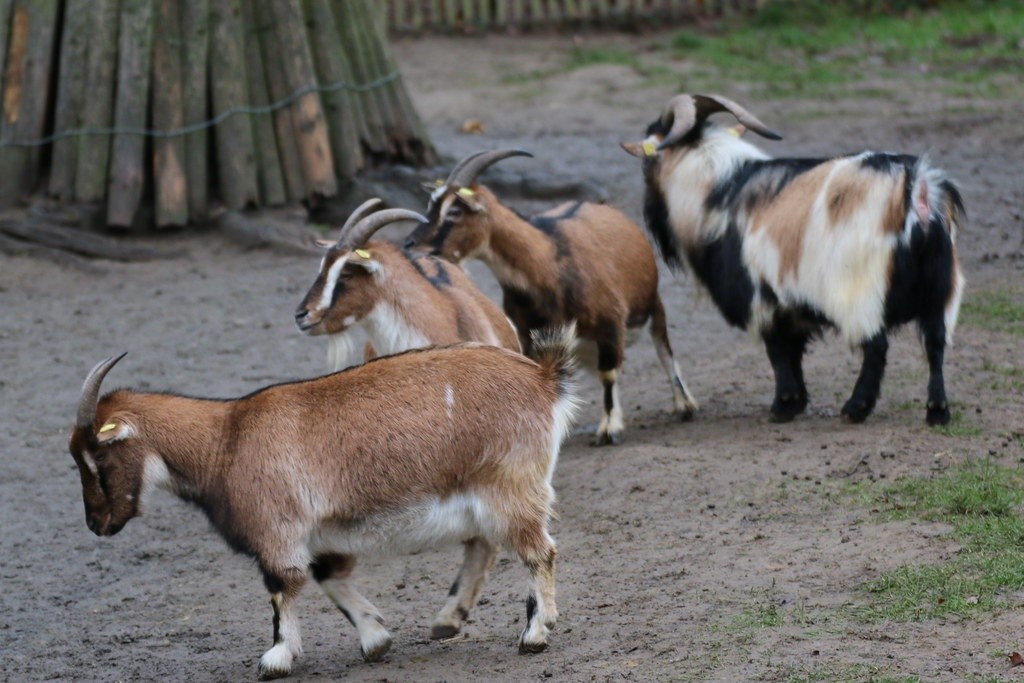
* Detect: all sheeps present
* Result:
[618,94,969,428]
[402,146,701,447]
[71,319,590,674]
[294,196,526,376]
